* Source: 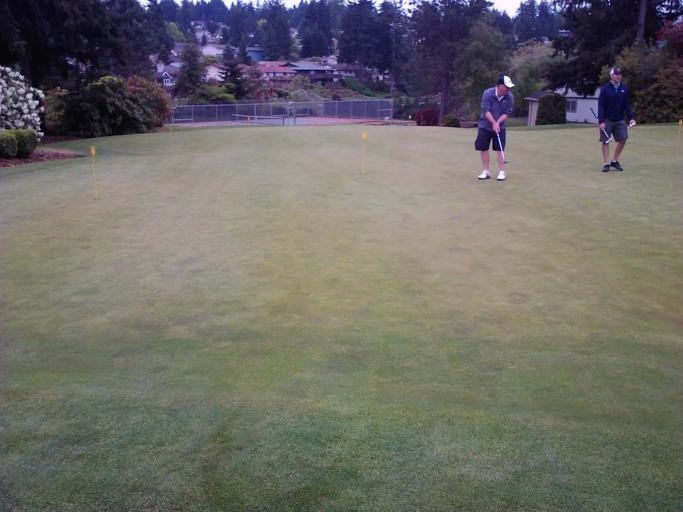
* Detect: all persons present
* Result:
[475,75,514,181]
[598,65,637,172]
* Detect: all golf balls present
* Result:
[630,123,635,128]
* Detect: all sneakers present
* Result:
[602,164,611,172]
[478,171,491,179]
[497,171,506,181]
[611,161,623,171]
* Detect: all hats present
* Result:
[610,66,622,76]
[497,76,515,88]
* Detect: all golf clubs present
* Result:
[497,131,508,163]
[590,108,611,144]
